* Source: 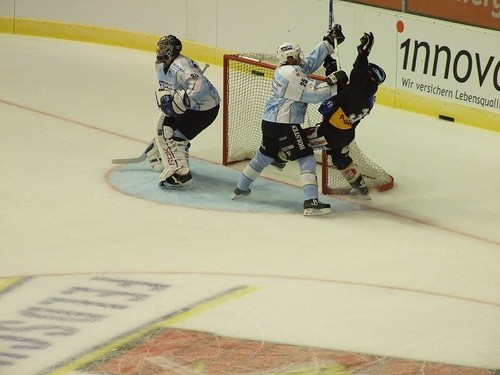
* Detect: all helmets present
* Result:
[364,63,386,84]
[155,35,182,64]
[276,42,301,65]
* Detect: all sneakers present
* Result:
[232,187,251,200]
[304,198,331,216]
[349,179,372,200]
[272,159,286,171]
[162,173,193,190]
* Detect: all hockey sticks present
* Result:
[332,13,340,72]
[112,64,209,164]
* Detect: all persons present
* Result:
[270,32,387,203]
[153,33,222,190]
[233,23,346,217]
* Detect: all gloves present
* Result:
[357,32,374,56]
[325,70,348,86]
[323,24,345,48]
